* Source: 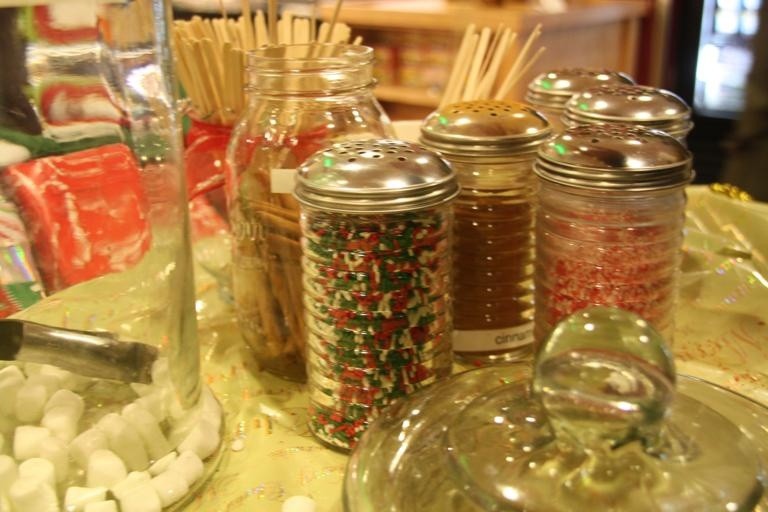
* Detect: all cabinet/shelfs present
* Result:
[309,0,652,119]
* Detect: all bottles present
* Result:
[523,67,636,132]
[225,44,400,383]
[559,84,694,138]
[294,139,459,456]
[0,0,224,512]
[531,123,695,362]
[416,99,555,370]
[342,306,768,512]
